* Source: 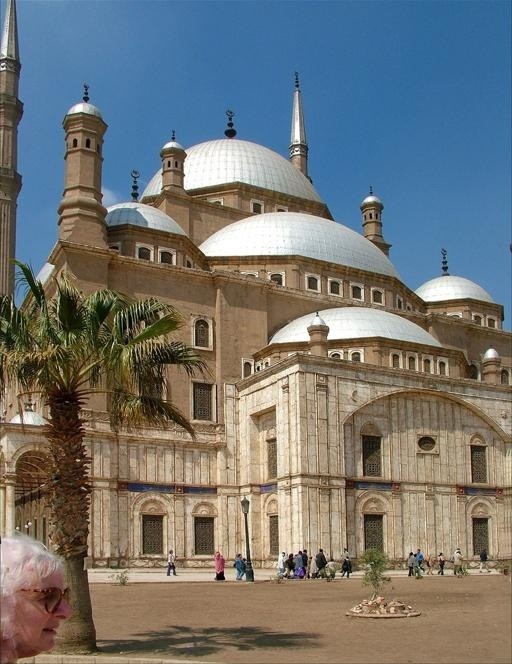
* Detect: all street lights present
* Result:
[237,490,255,581]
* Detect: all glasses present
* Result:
[16,583,73,615]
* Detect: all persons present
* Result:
[1,530,73,663]
[277,547,353,578]
[167,549,178,577]
[234,553,246,580]
[478,548,492,573]
[407,547,465,577]
[214,551,226,580]
